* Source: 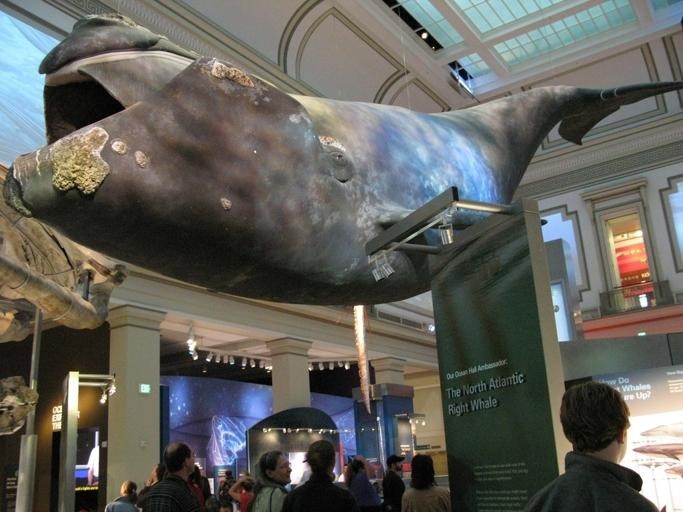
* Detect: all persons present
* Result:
[525,380,658,512]
[87,444,99,487]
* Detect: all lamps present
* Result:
[306,360,351,372]
[206,352,264,369]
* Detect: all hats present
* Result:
[385,454,405,466]
